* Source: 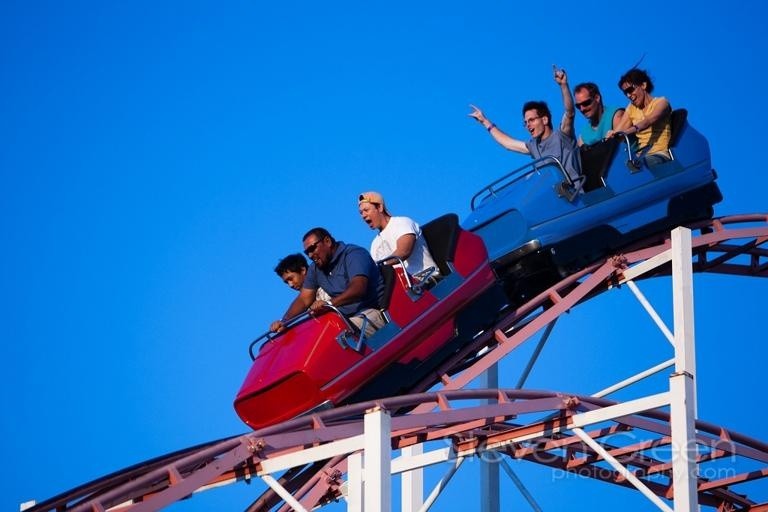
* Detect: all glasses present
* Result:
[575,94,595,109]
[304,237,325,255]
[624,86,637,96]
[523,116,543,129]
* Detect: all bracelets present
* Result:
[631,125,640,134]
[487,123,497,134]
[325,301,333,306]
[382,260,388,265]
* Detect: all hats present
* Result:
[358,192,391,216]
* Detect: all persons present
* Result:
[357,190,441,291]
[601,68,673,168]
[269,227,387,340]
[466,63,587,194]
[572,82,627,154]
[273,251,333,303]
